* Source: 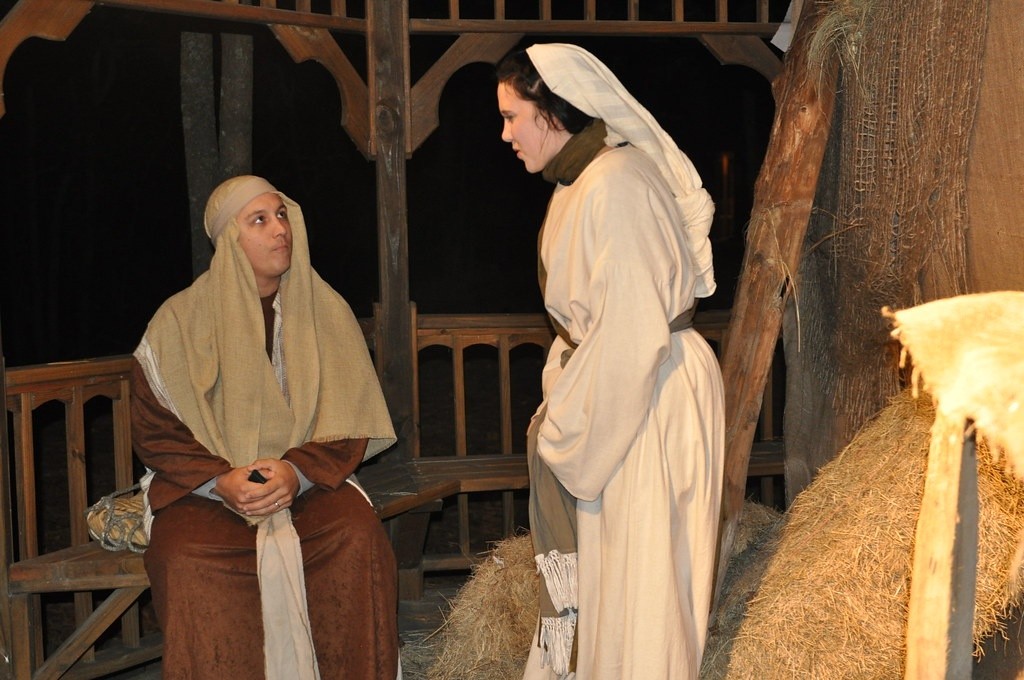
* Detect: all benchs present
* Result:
[9,441,785,680]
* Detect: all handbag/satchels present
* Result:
[82,481,148,555]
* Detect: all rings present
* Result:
[275,502,280,507]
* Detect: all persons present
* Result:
[496,43,726,680]
[129,175,403,680]
